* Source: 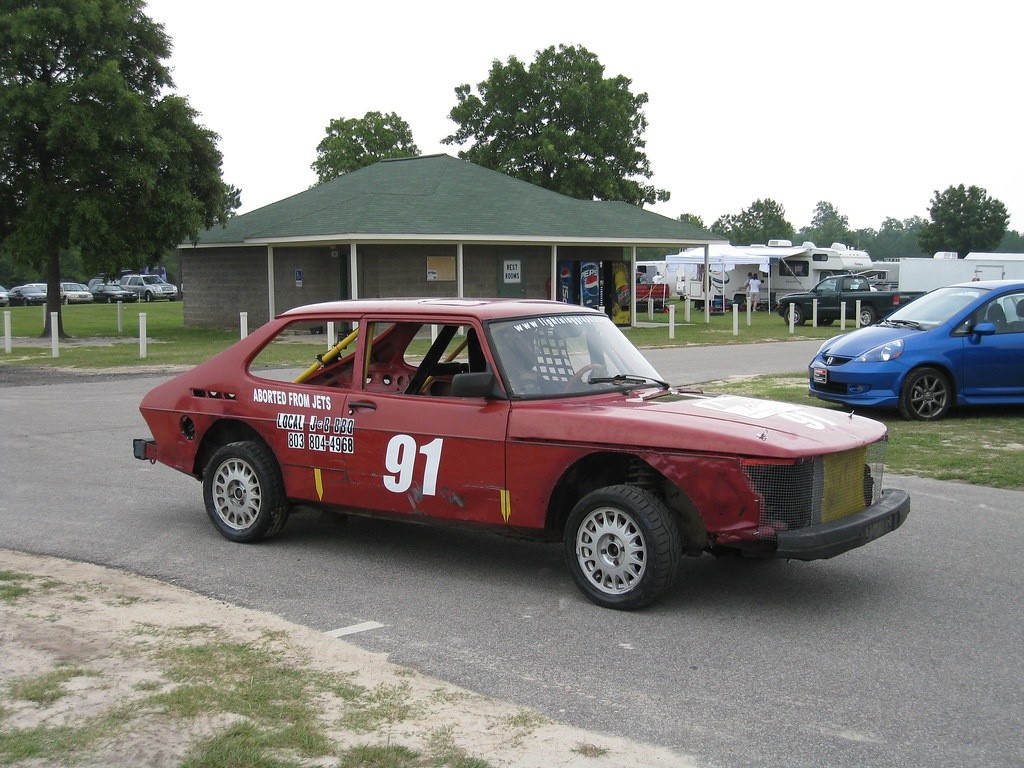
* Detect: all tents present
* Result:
[663,244,771,313]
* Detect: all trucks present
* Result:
[635,239,1024,312]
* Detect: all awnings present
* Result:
[740,247,811,291]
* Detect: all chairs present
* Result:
[1010,299,1024,332]
[467,328,486,372]
[734,293,748,304]
[987,303,1008,334]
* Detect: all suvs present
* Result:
[119,274,178,303]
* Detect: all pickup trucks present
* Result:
[777,274,928,328]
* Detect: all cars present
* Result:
[807,280,1024,421]
[23,283,47,293]
[8,286,47,307]
[89,284,138,304]
[0,286,10,307]
[59,277,121,305]
[132,297,915,612]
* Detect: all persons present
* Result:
[652,272,665,284]
[498,336,609,393]
[743,271,762,312]
